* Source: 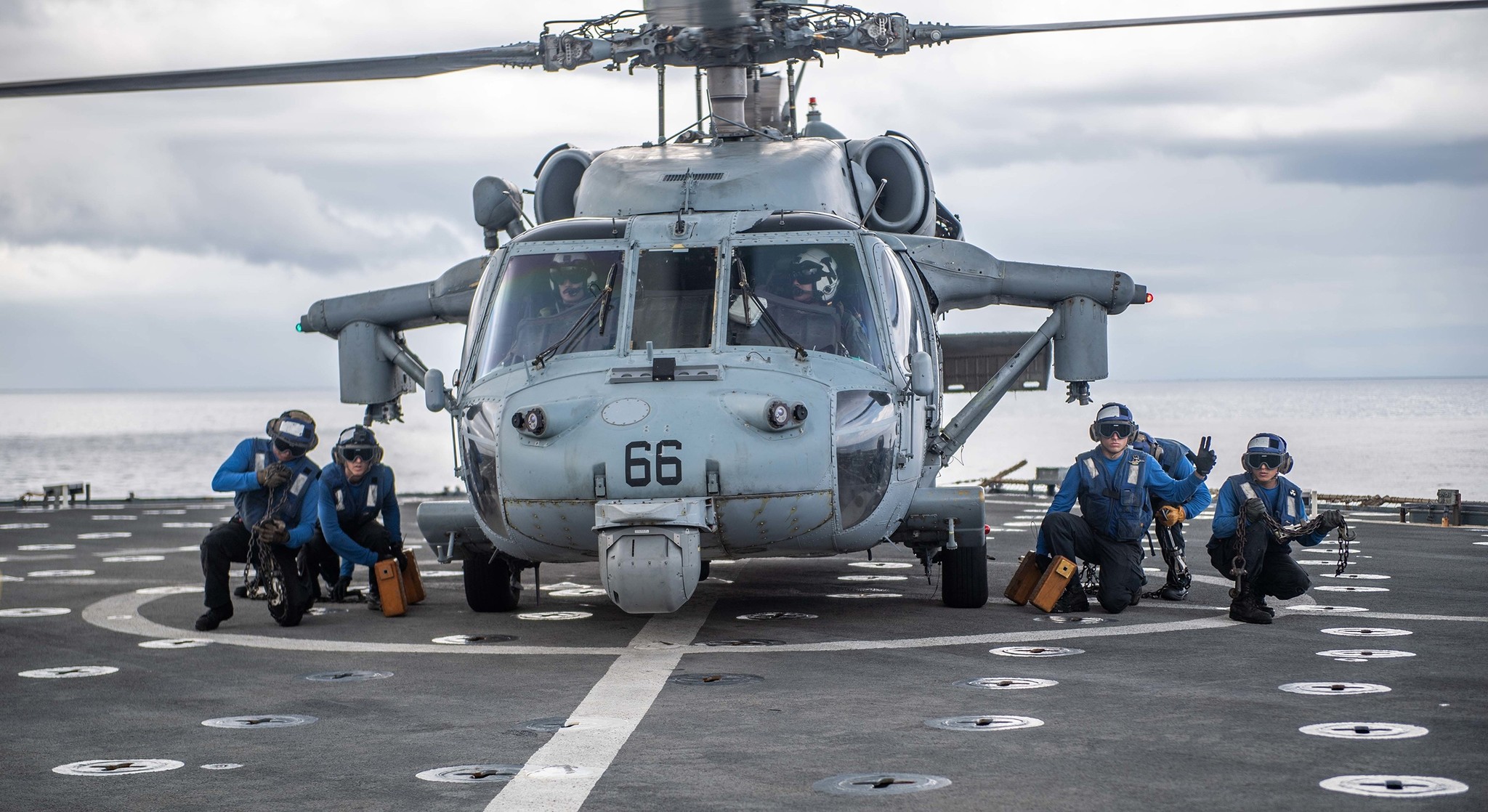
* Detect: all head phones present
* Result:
[1241,432,1294,475]
[1132,432,1164,463]
[1089,402,1140,445]
[331,424,384,467]
[266,410,319,451]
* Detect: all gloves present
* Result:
[257,458,292,488]
[1033,554,1053,571]
[1155,504,1186,528]
[1244,498,1265,524]
[377,549,408,571]
[1188,435,1217,477]
[251,522,289,545]
[1316,509,1342,535]
[331,576,352,602]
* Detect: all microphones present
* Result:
[791,287,815,295]
[568,283,584,296]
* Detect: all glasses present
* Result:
[271,434,307,458]
[548,265,592,285]
[338,446,377,462]
[1096,420,1134,439]
[789,264,820,280]
[1245,452,1285,470]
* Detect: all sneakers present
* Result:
[1228,581,1272,625]
[1159,579,1191,601]
[1128,584,1143,606]
[1254,587,1275,618]
[195,580,383,631]
[1140,566,1149,587]
[1049,578,1090,613]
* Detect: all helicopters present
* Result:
[2,2,1488,618]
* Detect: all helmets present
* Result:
[790,248,842,303]
[1246,433,1287,456]
[1128,431,1158,456]
[272,410,316,449]
[549,252,598,297]
[1094,402,1135,424]
[336,424,379,449]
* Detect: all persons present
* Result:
[195,410,322,631]
[1034,403,1216,612]
[1205,432,1342,624]
[535,253,602,318]
[786,248,875,365]
[1128,431,1212,601]
[294,425,407,611]
